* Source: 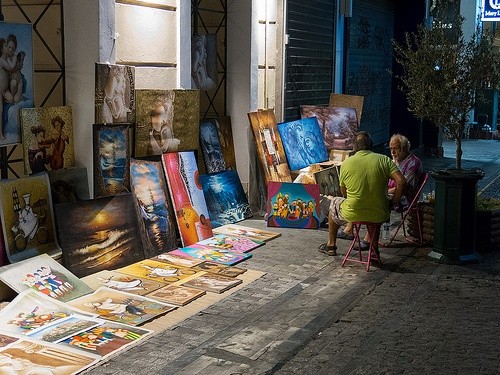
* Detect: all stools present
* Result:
[341,222,384,272]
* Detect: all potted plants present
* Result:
[406,191,500,245]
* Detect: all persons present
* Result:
[337,134,424,251]
[318,130,408,262]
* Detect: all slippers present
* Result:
[352,239,371,251]
[337,229,355,240]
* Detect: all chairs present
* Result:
[442,114,499,141]
[378,171,429,248]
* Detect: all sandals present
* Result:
[316,242,338,255]
